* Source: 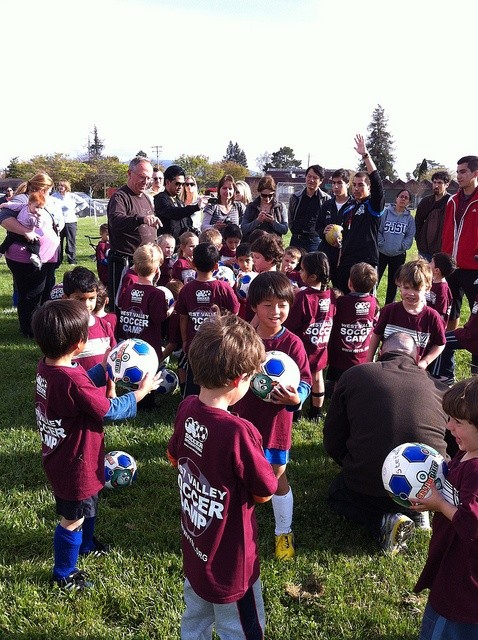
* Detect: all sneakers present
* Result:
[372,512,415,556]
[81,538,110,558]
[49,571,94,591]
[413,511,429,529]
[275,532,294,562]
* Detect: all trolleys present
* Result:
[74,192,106,217]
[85,235,102,262]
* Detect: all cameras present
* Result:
[208,196,220,205]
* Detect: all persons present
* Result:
[0,171,61,335]
[406,375,478,639]
[178,174,203,231]
[201,175,247,233]
[230,245,254,321]
[95,224,116,284]
[416,172,454,258]
[447,278,477,373]
[235,232,285,321]
[223,224,242,251]
[370,256,446,369]
[169,312,278,640]
[331,261,378,394]
[158,235,176,257]
[288,164,336,252]
[159,165,211,250]
[145,169,165,206]
[379,190,416,305]
[342,134,384,290]
[235,272,313,563]
[239,175,288,242]
[31,298,164,592]
[286,252,338,418]
[172,231,201,280]
[315,170,359,267]
[280,247,302,276]
[0,190,46,269]
[116,243,167,363]
[175,243,240,351]
[429,252,455,377]
[234,179,254,203]
[443,151,477,334]
[1,188,13,203]
[323,331,451,557]
[64,265,116,371]
[108,156,164,312]
[47,179,88,267]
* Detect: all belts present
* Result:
[298,234,318,239]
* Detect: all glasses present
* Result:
[172,182,185,186]
[259,191,274,198]
[184,182,194,186]
[153,177,162,179]
[132,171,150,181]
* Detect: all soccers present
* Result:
[103,451,137,489]
[249,350,300,402]
[156,369,179,395]
[325,225,343,247]
[107,338,159,391]
[382,442,448,509]
[156,286,174,309]
[236,272,259,302]
[212,265,236,288]
